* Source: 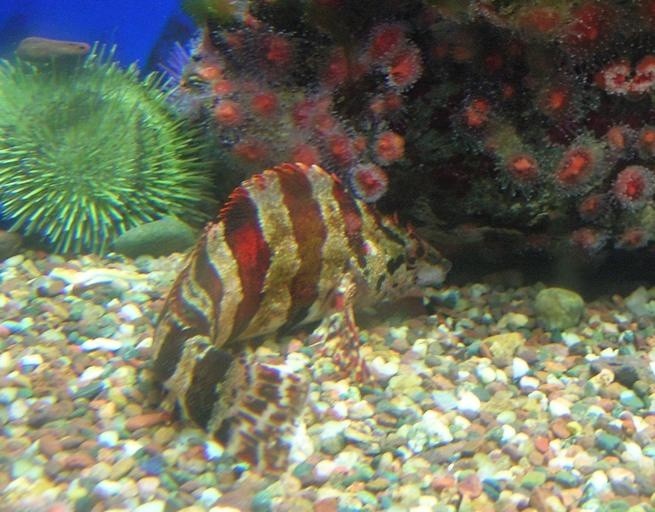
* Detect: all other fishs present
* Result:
[145,163,456,478]
[17,36,91,62]
